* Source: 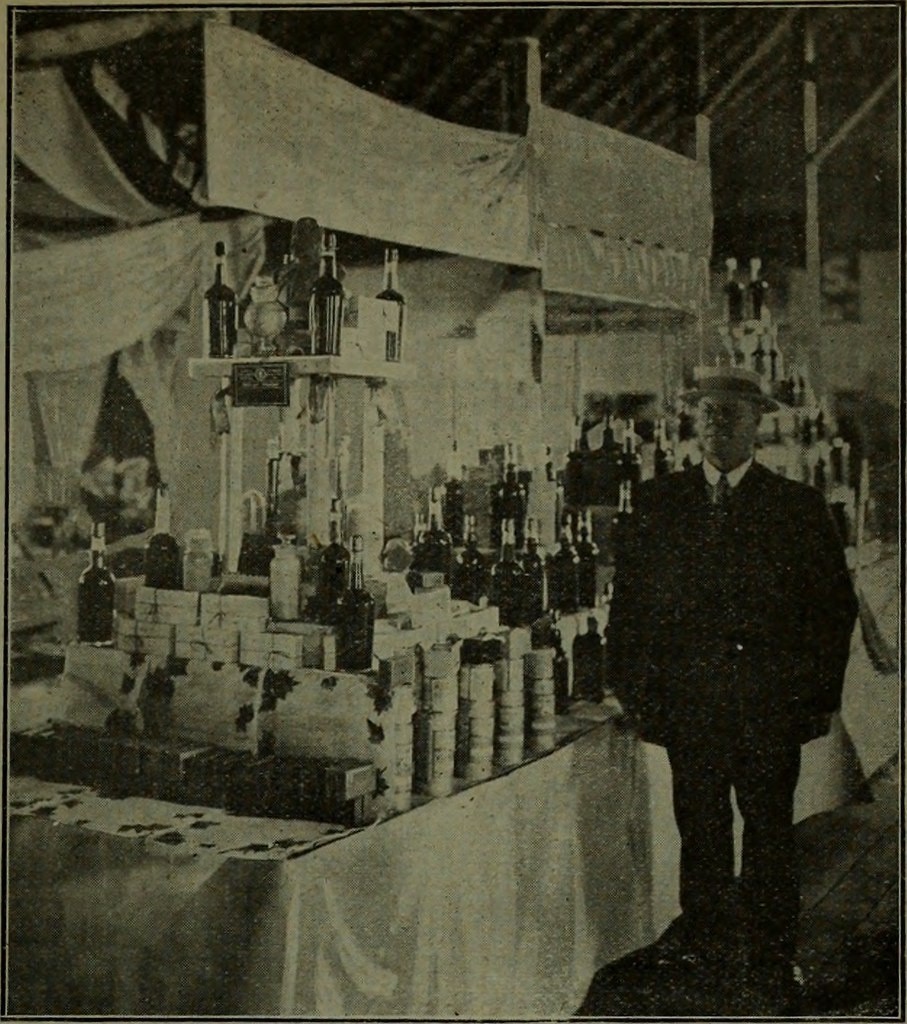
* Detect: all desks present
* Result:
[6,540,898,1019]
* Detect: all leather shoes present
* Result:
[754,934,804,1001]
[678,924,730,990]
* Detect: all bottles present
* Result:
[62,218,885,784]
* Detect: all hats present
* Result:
[676,368,780,413]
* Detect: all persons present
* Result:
[599,352,859,1013]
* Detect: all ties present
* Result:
[711,475,729,534]
